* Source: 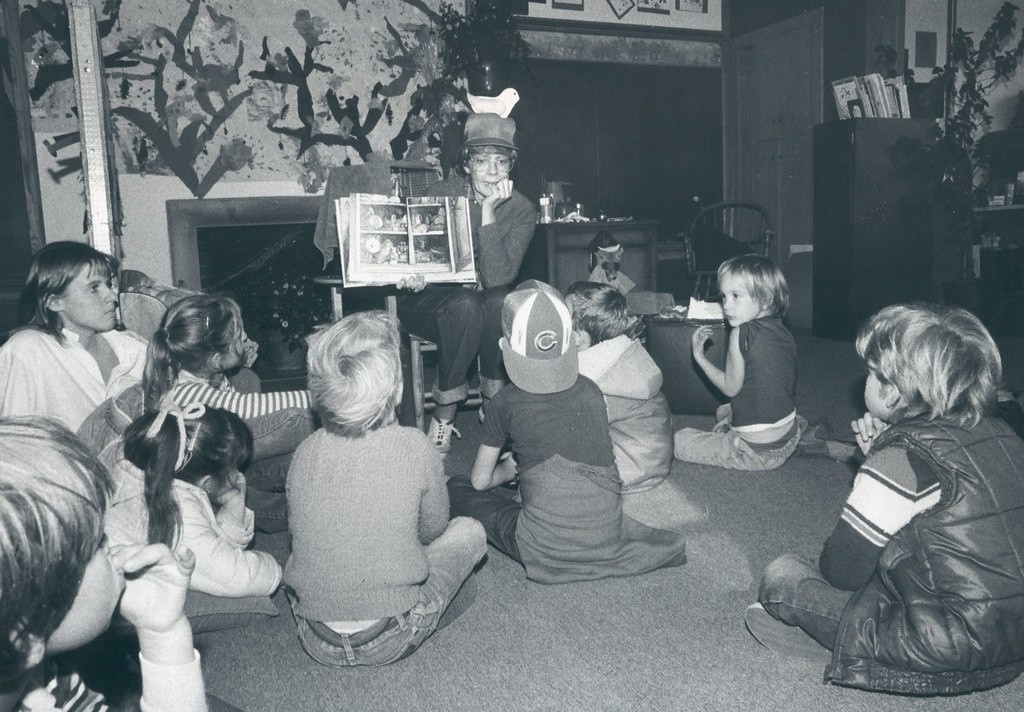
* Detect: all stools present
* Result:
[411,334,485,434]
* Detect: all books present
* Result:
[334,192,476,289]
[832,74,911,118]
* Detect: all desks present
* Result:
[309,277,400,325]
[520,221,660,299]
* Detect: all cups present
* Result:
[557,202,568,218]
[573,202,584,222]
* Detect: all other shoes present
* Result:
[745,602,834,665]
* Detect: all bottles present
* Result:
[539,184,555,224]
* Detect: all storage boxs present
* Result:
[643,315,732,417]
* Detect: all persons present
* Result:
[564,281,673,493]
[396,114,539,455]
[673,253,866,472]
[0,242,325,635]
[445,279,688,585]
[744,301,1023,697]
[282,308,489,666]
[0,413,242,712]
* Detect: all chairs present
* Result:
[683,199,774,305]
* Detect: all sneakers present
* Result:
[427,414,461,460]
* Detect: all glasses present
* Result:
[469,152,515,172]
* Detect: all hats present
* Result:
[464,113,519,150]
[501,279,578,395]
